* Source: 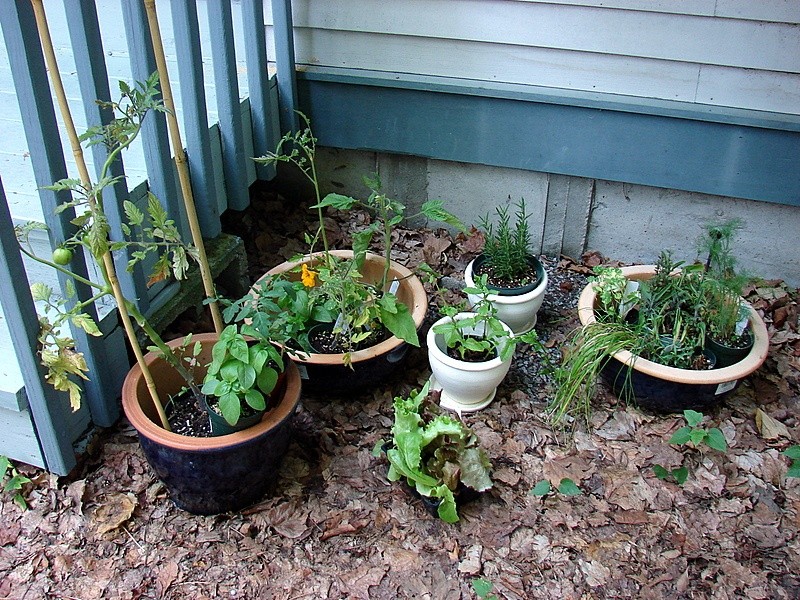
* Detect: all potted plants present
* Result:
[540,216,777,444]
[419,262,556,379]
[471,196,543,296]
[200,323,286,436]
[14,70,215,439]
[585,263,639,331]
[203,268,309,404]
[651,317,717,370]
[205,109,477,372]
[692,219,777,369]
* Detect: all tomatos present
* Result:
[52,248,73,265]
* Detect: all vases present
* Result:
[121,331,302,517]
[426,313,515,406]
[464,260,549,334]
[242,250,427,400]
